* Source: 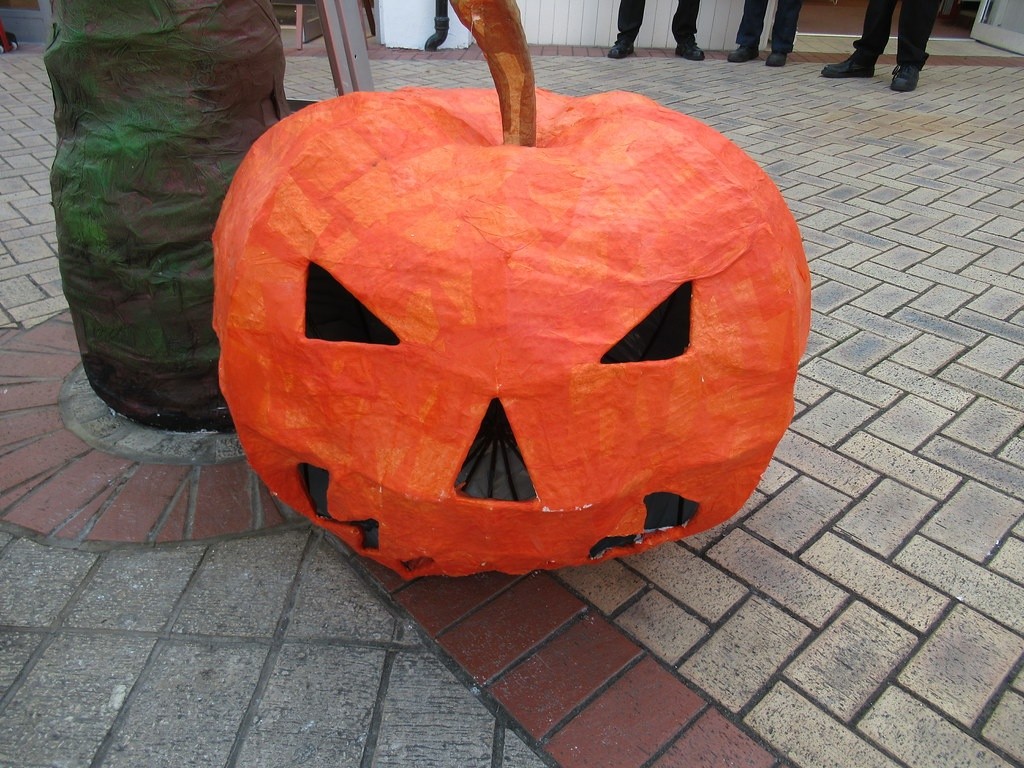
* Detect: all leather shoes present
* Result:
[891,64,920,91]
[675,35,704,60]
[608,40,634,59]
[821,53,874,78]
[766,52,787,66]
[728,46,759,62]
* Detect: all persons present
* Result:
[608,0,705,60]
[728,0,802,67]
[821,0,943,92]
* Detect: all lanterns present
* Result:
[211,80,812,582]
